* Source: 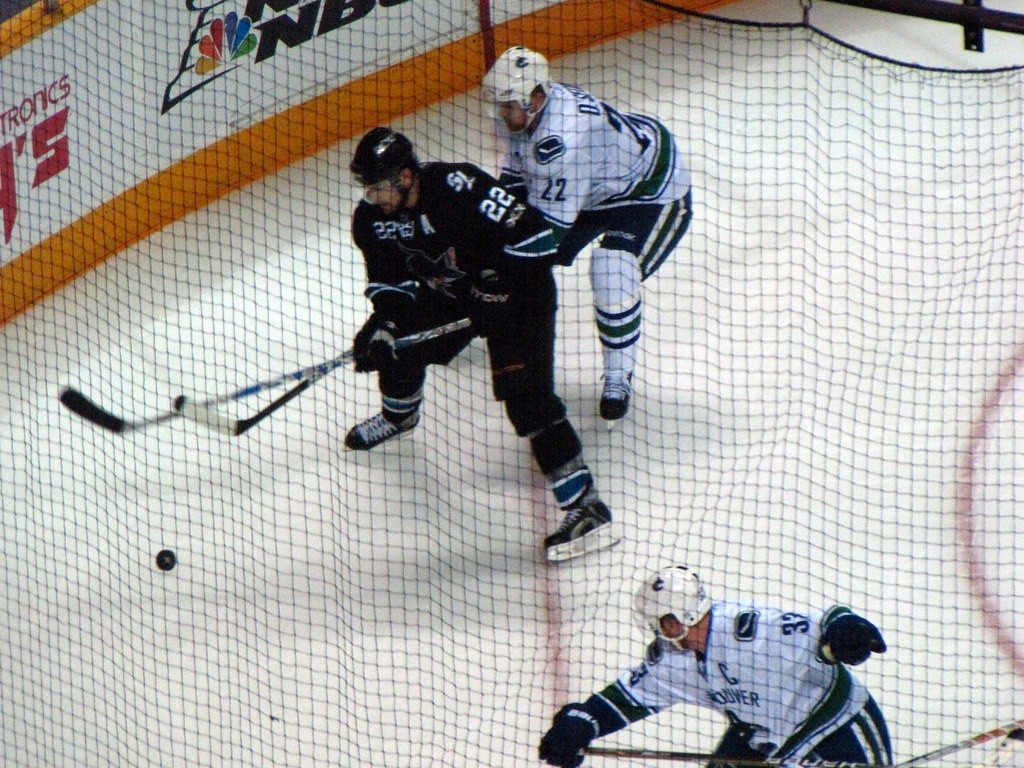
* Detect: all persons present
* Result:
[538,565,893,768]
[480,46,692,419]
[344,126,612,549]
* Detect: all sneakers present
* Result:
[544,482,621,561]
[601,372,633,431]
[341,403,420,452]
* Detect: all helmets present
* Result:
[349,126,412,187]
[635,565,713,627]
[482,46,549,108]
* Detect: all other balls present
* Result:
[156,549,177,571]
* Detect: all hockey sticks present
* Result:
[173,348,355,437]
[582,744,886,767]
[894,718,1024,768]
[55,315,475,436]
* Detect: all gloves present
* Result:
[822,609,886,668]
[353,312,399,373]
[539,704,610,768]
[470,264,520,337]
